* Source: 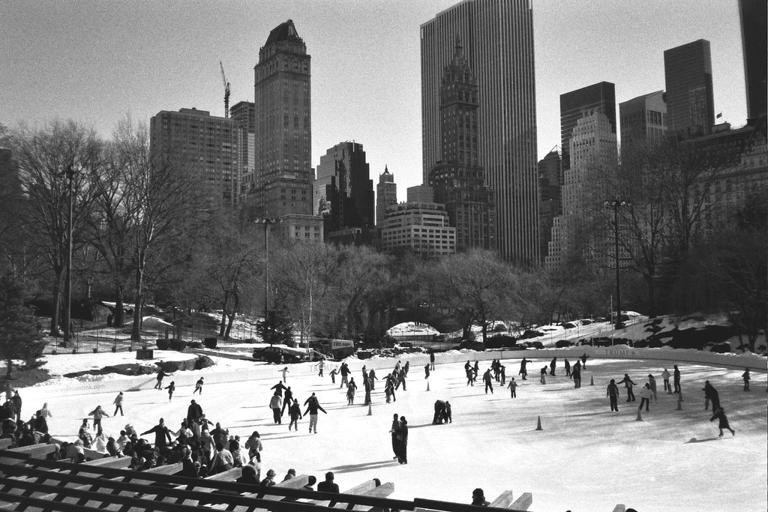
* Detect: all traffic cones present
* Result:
[678,390,684,401]
[635,408,642,422]
[366,401,373,415]
[589,375,595,386]
[667,383,673,394]
[534,415,544,431]
[426,381,431,392]
[674,400,684,411]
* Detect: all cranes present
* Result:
[216,58,232,118]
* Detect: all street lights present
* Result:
[253,216,284,324]
[609,294,614,345]
[603,197,626,325]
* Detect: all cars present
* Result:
[253,338,354,364]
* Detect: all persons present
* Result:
[390,414,408,464]
[194,377,204,395]
[165,381,175,400]
[472,488,491,505]
[269,367,327,433]
[0,390,53,446]
[154,368,170,390]
[465,349,593,399]
[260,469,316,492]
[78,391,264,484]
[433,400,453,425]
[315,358,410,406]
[424,352,435,380]
[607,364,735,438]
[318,472,340,493]
[741,368,750,392]
